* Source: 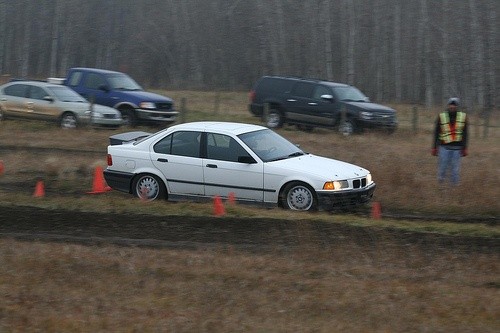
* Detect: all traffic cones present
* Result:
[33,181,46,197]
[212,196,224,214]
[226,192,236,204]
[371,203,381,218]
[88,166,105,194]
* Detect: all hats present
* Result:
[447,97,460,106]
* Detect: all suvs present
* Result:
[248,76,398,138]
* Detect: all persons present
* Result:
[431,98,468,191]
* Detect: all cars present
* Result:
[102,121,376,213]
[0,81,123,130]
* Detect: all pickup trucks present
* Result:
[9,67,178,130]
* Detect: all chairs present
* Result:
[159,140,201,156]
